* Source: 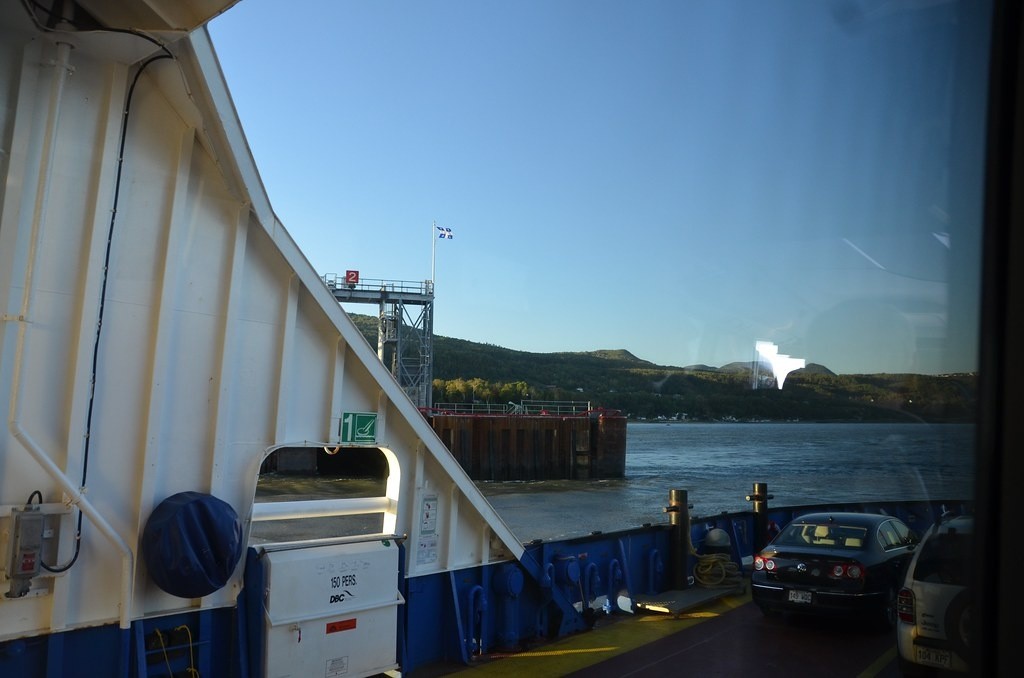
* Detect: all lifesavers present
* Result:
[767,522,781,540]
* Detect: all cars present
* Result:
[750,512,921,635]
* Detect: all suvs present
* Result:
[894,510,981,678]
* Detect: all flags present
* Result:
[436,227,453,239]
